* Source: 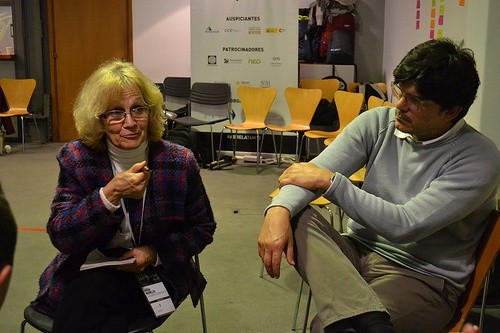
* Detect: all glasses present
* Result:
[390,81,434,111]
[101,106,150,119]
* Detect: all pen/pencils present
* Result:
[143,166,152,175]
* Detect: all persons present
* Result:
[256,38,500,333]
[0,196,18,308]
[30,60,217,333]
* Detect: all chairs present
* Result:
[0,77,500,333]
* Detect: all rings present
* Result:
[141,180,149,187]
